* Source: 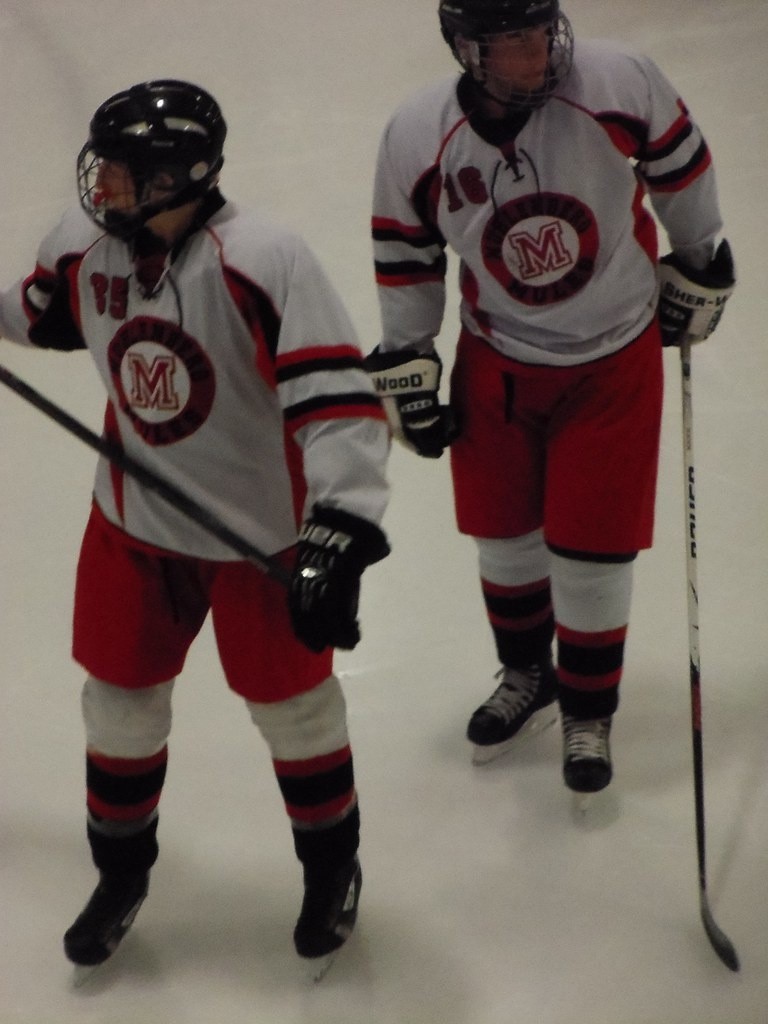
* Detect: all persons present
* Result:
[367,0,736,822]
[0,77,392,995]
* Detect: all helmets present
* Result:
[75,80,226,241]
[438,0,574,107]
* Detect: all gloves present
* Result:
[365,343,462,458]
[657,234,735,347]
[283,504,390,653]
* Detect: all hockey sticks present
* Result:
[1,367,365,650]
[679,341,742,975]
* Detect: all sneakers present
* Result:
[562,714,616,814]
[293,853,362,980]
[468,650,558,766]
[64,860,151,986]
